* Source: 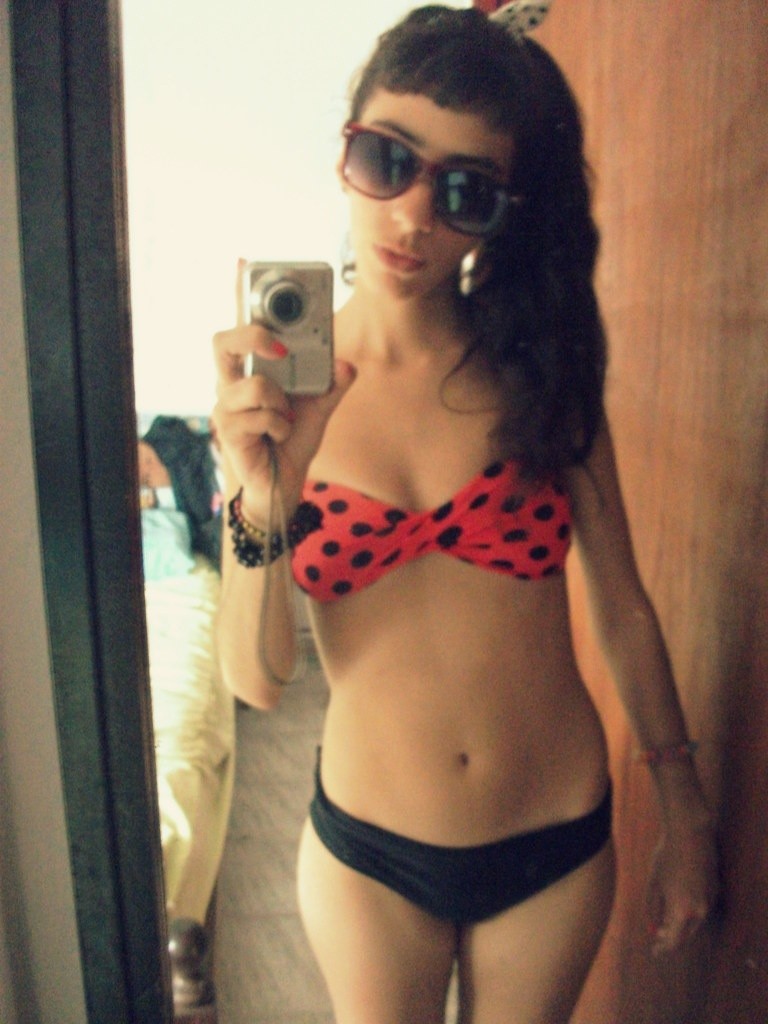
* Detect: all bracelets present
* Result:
[227,486,325,569]
[641,739,700,771]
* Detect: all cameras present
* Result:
[241,261,334,396]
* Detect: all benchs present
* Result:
[136,429,209,509]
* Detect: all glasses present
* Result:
[338,116,522,237]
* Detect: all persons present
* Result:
[218,0,717,1024]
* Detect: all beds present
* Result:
[133,508,236,1023]
